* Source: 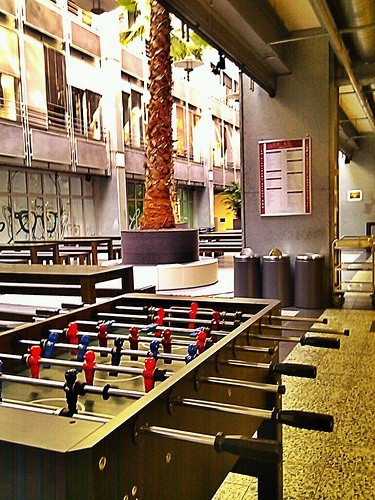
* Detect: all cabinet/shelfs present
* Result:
[331,235,375,307]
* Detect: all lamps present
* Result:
[67,0,202,81]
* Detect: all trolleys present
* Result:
[332,235,375,314]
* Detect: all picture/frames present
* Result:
[348,189,363,202]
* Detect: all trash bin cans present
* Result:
[232,247,261,298]
[292,253,330,309]
[260,248,293,308]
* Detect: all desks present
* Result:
[0,226,244,336]
